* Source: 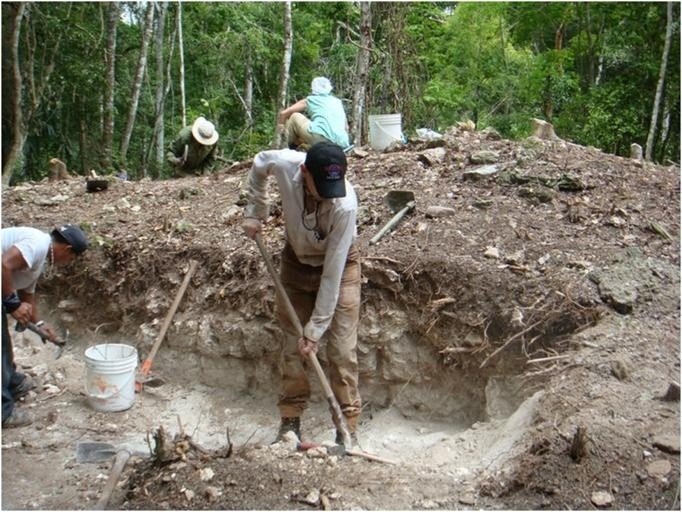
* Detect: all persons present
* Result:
[242,141,362,453]
[1,223,89,427]
[166,116,219,179]
[278,76,350,150]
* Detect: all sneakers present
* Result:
[271,418,300,444]
[335,431,364,452]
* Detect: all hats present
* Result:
[312,76,332,94]
[56,224,87,254]
[192,117,218,145]
[305,141,347,198]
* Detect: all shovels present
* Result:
[254,232,371,459]
[15,321,69,360]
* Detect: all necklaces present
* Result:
[43,240,54,280]
[301,178,320,231]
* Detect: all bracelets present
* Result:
[3,291,22,312]
[35,321,45,329]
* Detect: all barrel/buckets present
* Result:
[85,344,138,412]
[368,113,403,150]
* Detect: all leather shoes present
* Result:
[11,373,33,401]
[2,408,35,429]
